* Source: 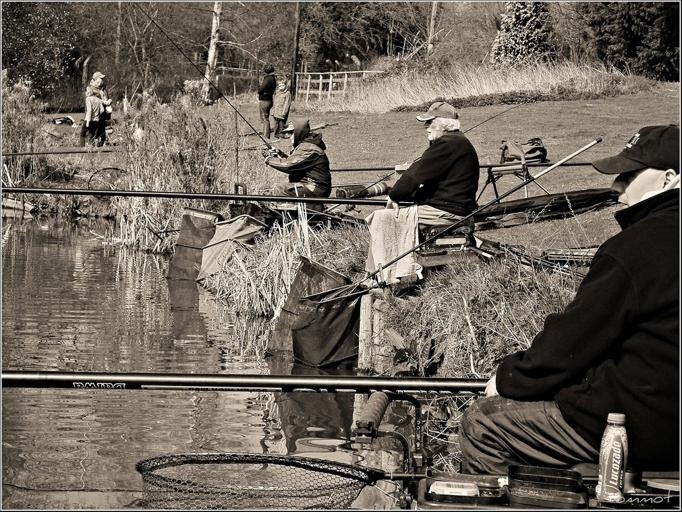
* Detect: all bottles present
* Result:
[595,412,630,504]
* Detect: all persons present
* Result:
[72,70,113,147]
[270,79,292,139]
[456,122,679,475]
[317,51,361,91]
[260,117,333,215]
[257,63,276,139]
[360,100,479,287]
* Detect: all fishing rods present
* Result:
[2,370,487,393]
[3,187,414,206]
[134,2,272,150]
[3,150,132,156]
[321,95,543,213]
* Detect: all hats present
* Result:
[92,72,105,80]
[592,124,679,175]
[415,101,459,121]
[282,122,294,132]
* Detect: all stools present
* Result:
[504,462,679,509]
[416,219,474,267]
[306,202,325,212]
